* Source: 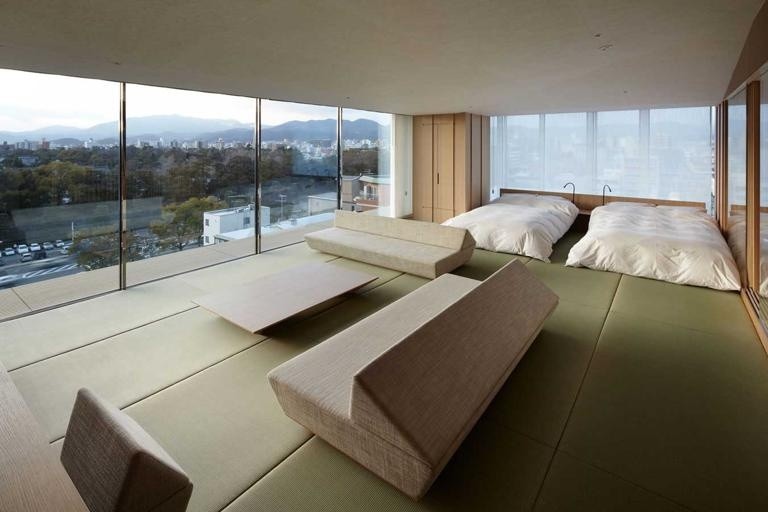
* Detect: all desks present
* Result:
[0,361,91,512]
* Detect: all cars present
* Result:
[0,239,76,265]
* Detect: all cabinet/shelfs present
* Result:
[413,113,490,223]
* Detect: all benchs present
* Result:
[267,259,560,502]
[306,208,475,279]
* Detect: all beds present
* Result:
[565,202,741,291]
[729,211,768,300]
[444,193,580,263]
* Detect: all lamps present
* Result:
[564,180,575,204]
[603,185,611,204]
